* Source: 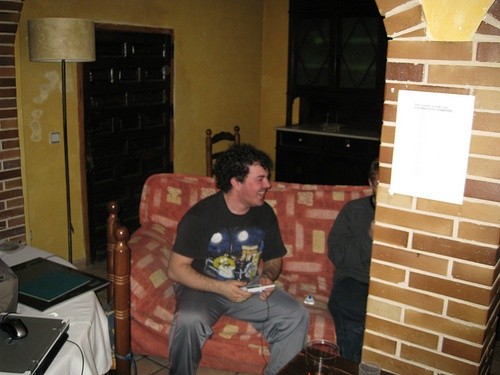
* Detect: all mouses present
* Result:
[0,317,29,339]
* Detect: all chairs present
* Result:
[205,124,242,178]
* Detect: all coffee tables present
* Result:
[276,344,394,375]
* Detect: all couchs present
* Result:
[99,162,375,375]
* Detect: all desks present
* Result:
[0,237,115,375]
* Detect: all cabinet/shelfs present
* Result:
[274,118,379,185]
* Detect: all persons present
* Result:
[326,158,380,363]
[166,141,311,375]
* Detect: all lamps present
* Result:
[24,14,97,261]
